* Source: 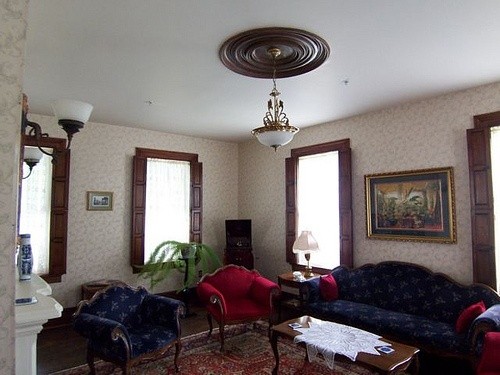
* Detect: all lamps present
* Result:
[292,230,321,277]
[20,147,42,182]
[22,95,96,156]
[251,49,299,152]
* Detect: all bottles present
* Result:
[18,233,33,279]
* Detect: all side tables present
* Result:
[278,272,322,315]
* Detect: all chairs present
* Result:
[199,264,279,351]
[73,282,184,375]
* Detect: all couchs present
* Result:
[299,261,500,375]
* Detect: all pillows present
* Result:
[456,300,486,332]
[319,274,338,302]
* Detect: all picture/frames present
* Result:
[364,166,458,245]
[87,192,114,211]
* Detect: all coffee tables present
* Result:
[268,316,420,375]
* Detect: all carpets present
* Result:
[49,319,377,375]
